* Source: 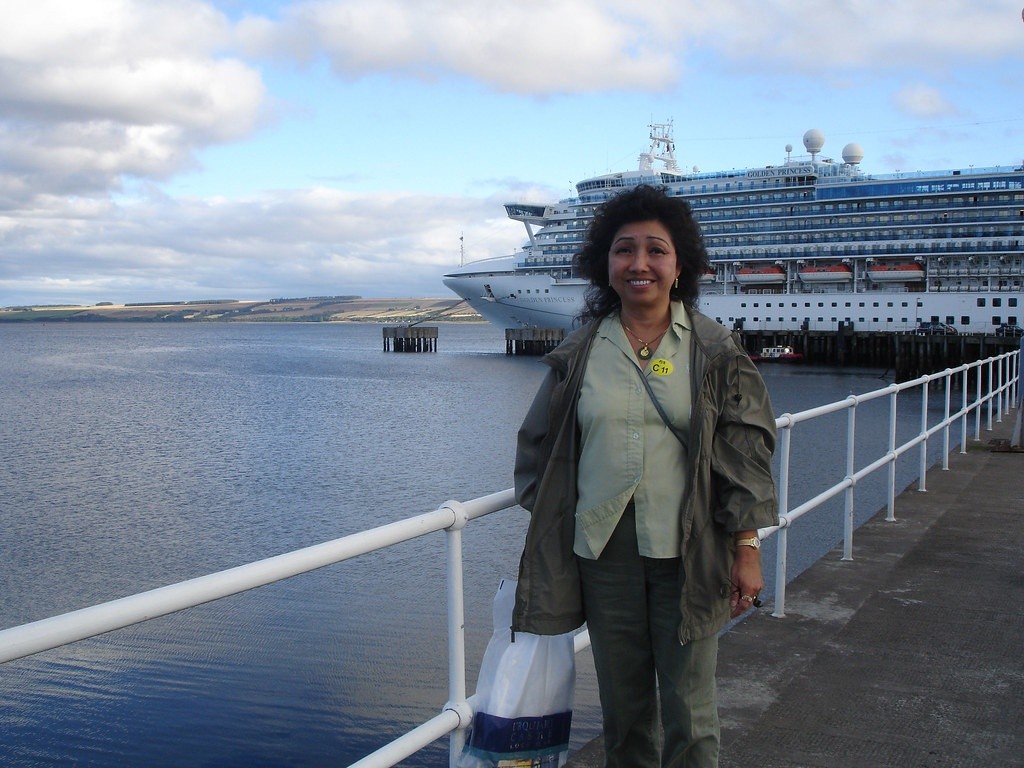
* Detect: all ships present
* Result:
[442,117,1021,360]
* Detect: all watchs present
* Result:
[736,537,761,550]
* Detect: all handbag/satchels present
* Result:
[456,579,577,768]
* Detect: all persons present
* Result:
[509,184,780,768]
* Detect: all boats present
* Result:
[748,344,804,364]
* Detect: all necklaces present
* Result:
[621,320,667,360]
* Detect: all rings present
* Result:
[754,596,756,600]
[741,595,753,603]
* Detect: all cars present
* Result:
[995,326,1024,338]
[913,321,959,336]
